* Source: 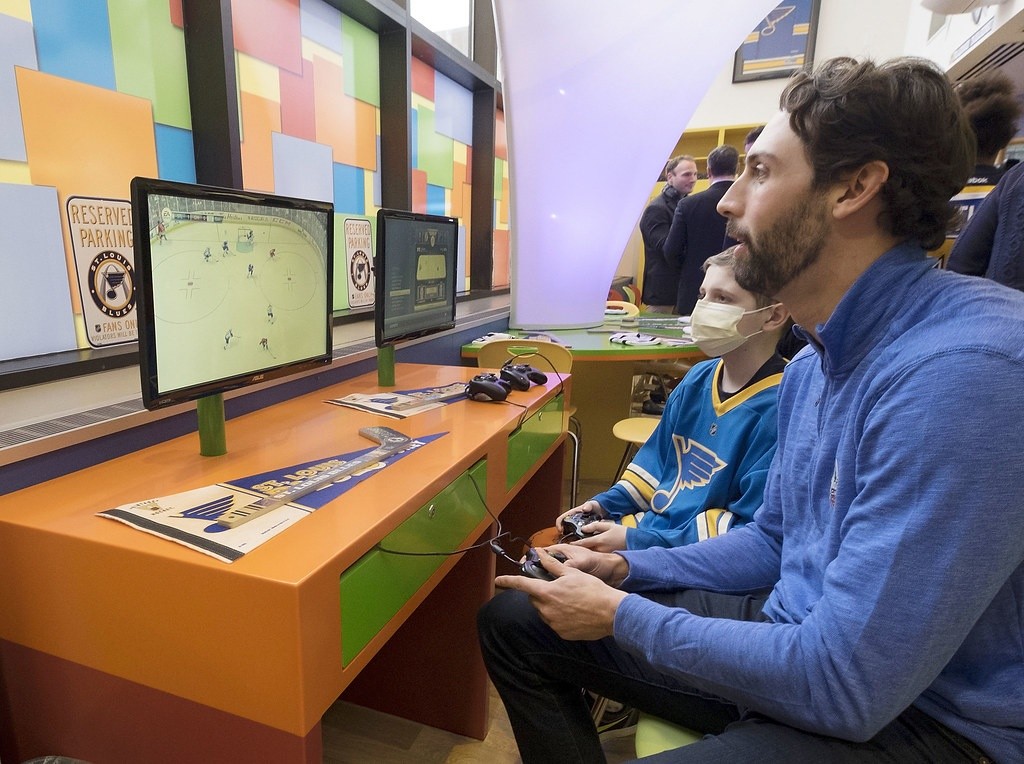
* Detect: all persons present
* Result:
[523,242,790,731]
[473,53,1024,763]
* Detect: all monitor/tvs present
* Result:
[374,207,458,347]
[129,175,333,412]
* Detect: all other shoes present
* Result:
[581,689,639,742]
[643,399,664,415]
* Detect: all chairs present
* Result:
[612,415,663,491]
[478,341,581,511]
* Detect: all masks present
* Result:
[690,298,776,359]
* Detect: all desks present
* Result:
[461,307,709,479]
[0,361,574,764]
